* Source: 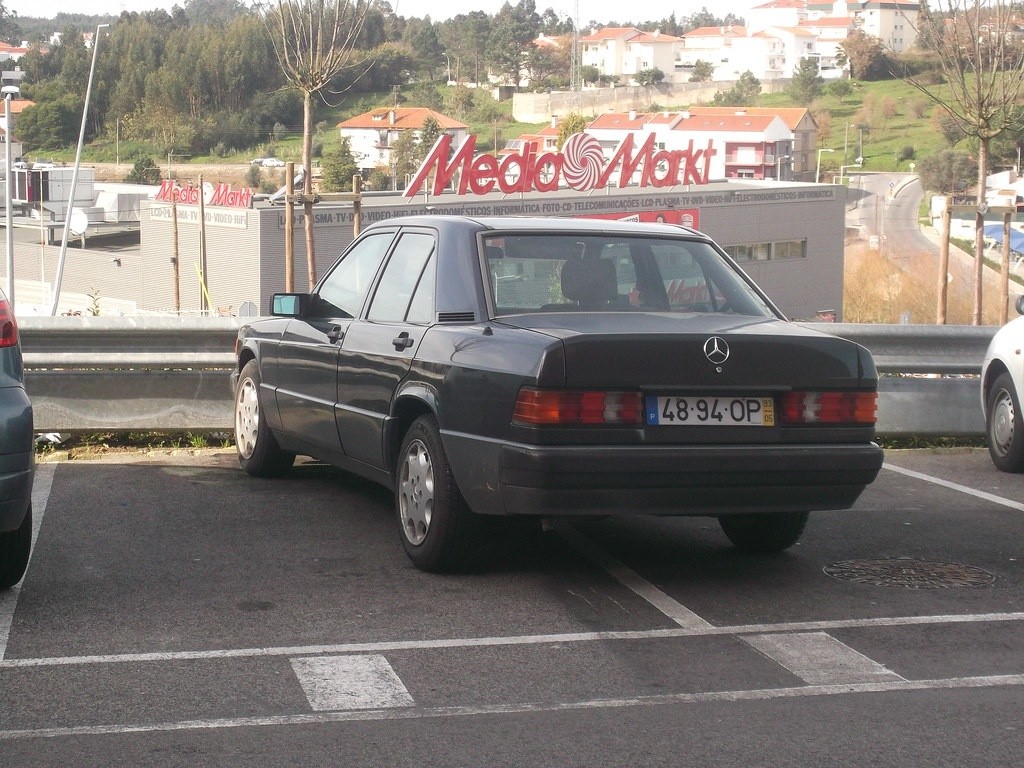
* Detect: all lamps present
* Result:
[114,258,118,263]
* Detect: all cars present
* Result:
[980,296,1024,475]
[227,213,885,573]
[1,284,37,587]
[249,157,285,167]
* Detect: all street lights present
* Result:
[843,122,854,176]
[441,51,451,81]
[816,147,835,183]
[0,85,19,310]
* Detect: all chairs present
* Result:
[540,257,623,312]
